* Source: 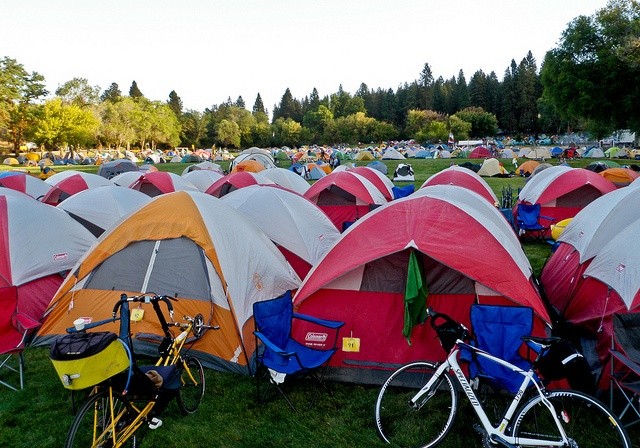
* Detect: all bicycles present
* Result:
[374,306,633,448]
[49,294,220,448]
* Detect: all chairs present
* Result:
[516,203,556,242]
[609,311,640,428]
[463,306,550,408]
[250,291,345,413]
[0,286,42,391]
[66,294,130,410]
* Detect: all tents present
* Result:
[542,187,640,328]
[561,220,640,414]
[585,162,624,176]
[601,169,639,189]
[0,171,118,206]
[302,172,389,234]
[118,168,224,198]
[182,162,224,176]
[320,163,333,175]
[3,142,235,167]
[510,167,619,245]
[29,192,305,386]
[302,163,329,181]
[37,166,55,180]
[417,164,503,214]
[54,187,153,239]
[267,135,640,161]
[259,168,313,198]
[408,184,547,305]
[0,188,98,354]
[207,172,279,200]
[219,185,343,282]
[229,161,266,175]
[517,161,552,179]
[289,160,304,177]
[346,167,396,203]
[479,157,511,179]
[293,198,561,401]
[368,160,389,176]
[98,159,138,181]
